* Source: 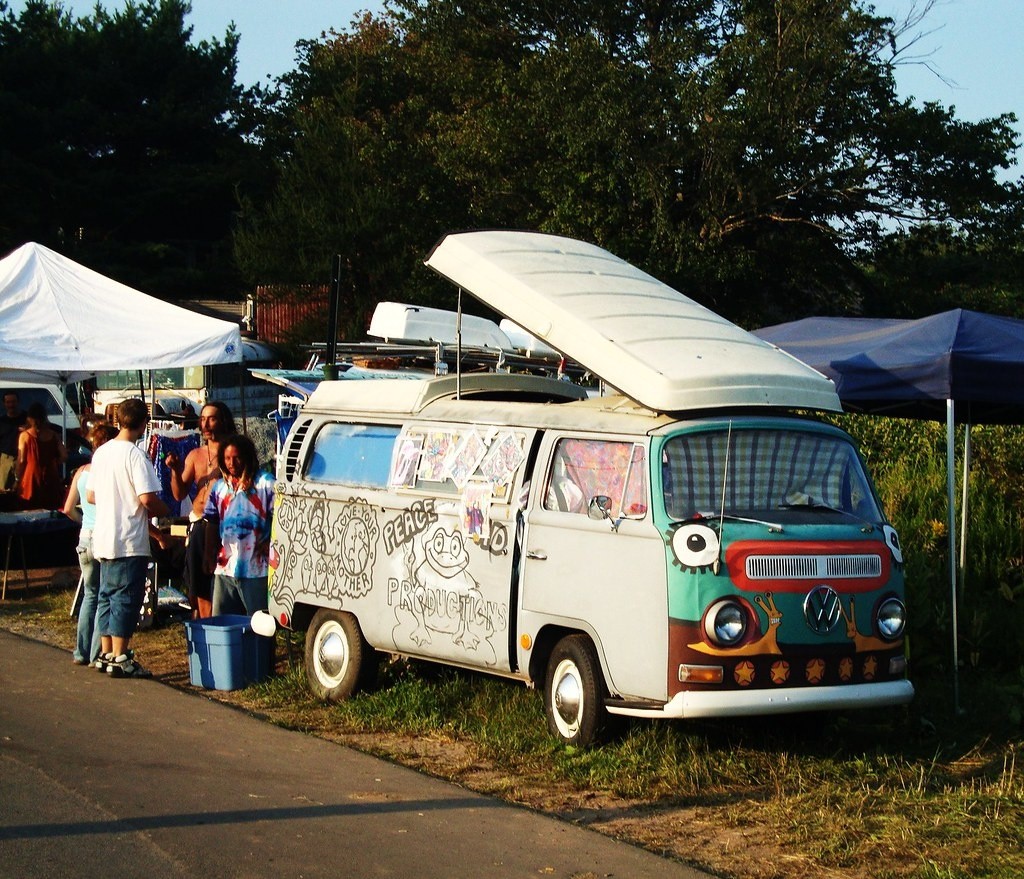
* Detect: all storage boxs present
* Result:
[184,615,276,690]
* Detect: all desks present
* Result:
[0,505,83,599]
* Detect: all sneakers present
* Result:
[96,652,113,673]
[106,650,153,679]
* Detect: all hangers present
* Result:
[151,419,195,439]
[267,394,294,420]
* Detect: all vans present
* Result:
[248,232,915,748]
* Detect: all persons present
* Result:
[166,403,277,621]
[12,404,69,511]
[0,389,25,460]
[63,398,166,678]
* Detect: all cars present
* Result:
[0,381,79,430]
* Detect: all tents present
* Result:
[0,241,243,614]
[746,308,1023,715]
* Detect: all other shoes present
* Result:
[74,659,90,666]
[87,659,98,667]
